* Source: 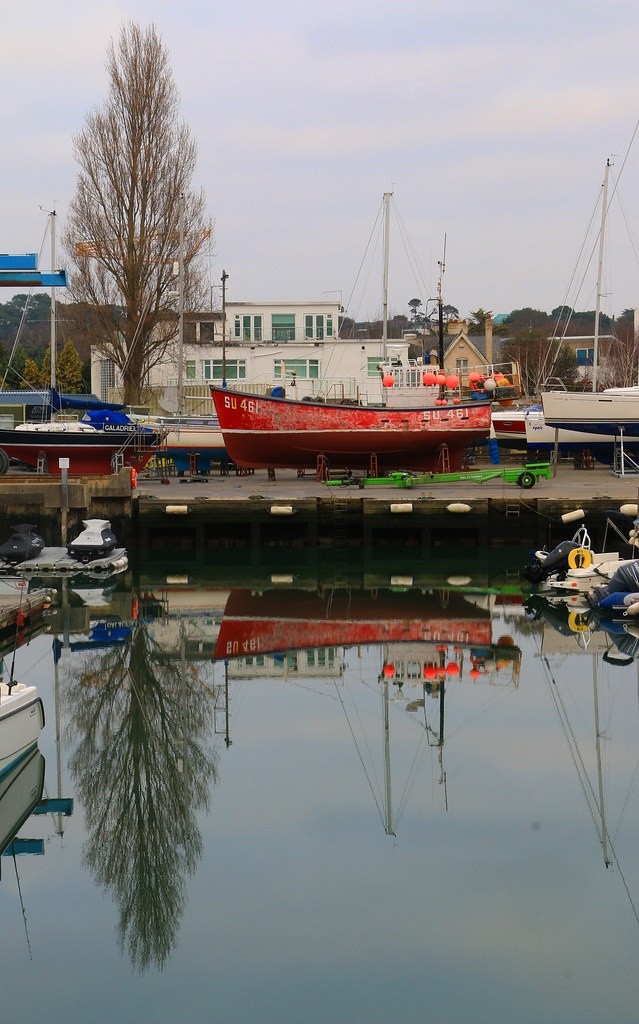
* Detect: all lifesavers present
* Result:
[567,547,591,571]
[567,611,591,634]
[130,469,136,491]
[132,598,140,618]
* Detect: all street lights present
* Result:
[221,273,229,390]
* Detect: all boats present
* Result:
[0,208,289,472]
[0,681,45,778]
[518,511,639,611]
[0,423,172,479]
[209,191,493,470]
[491,156,639,449]
